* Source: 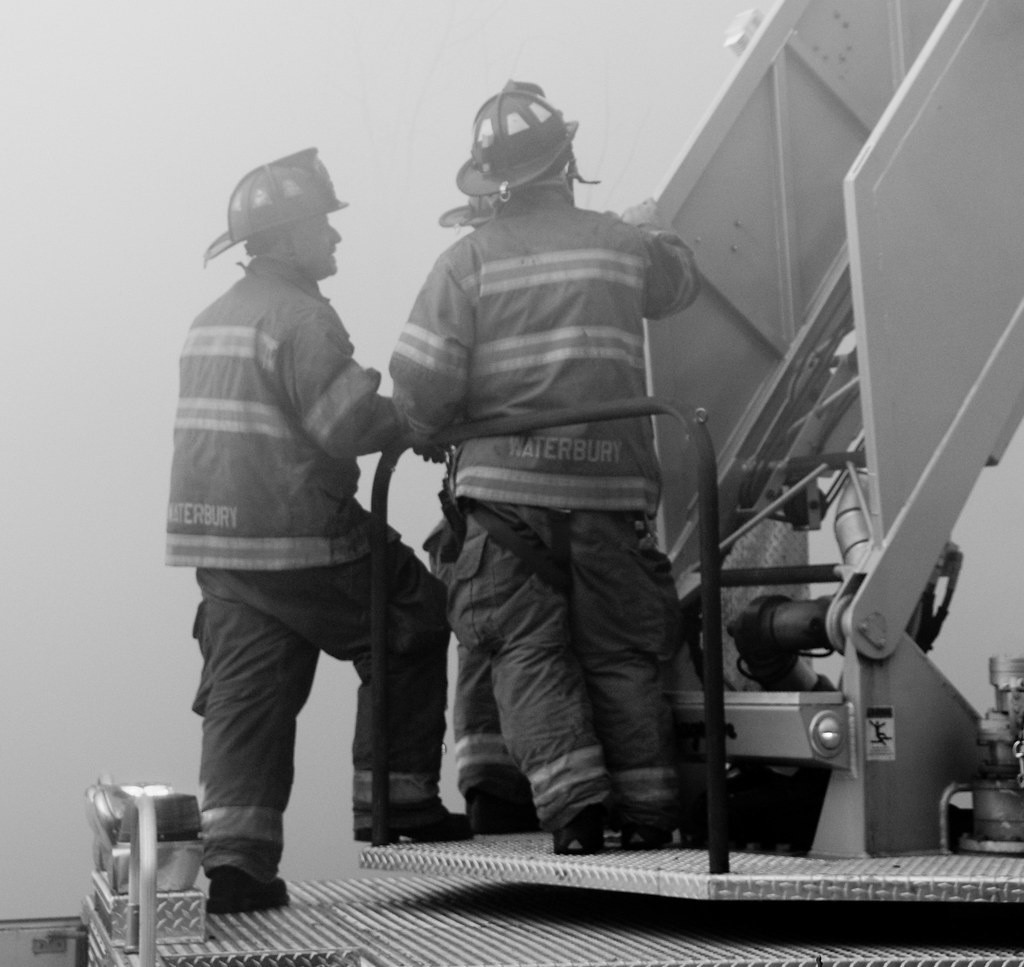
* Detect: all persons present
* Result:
[422,496,538,835]
[169,148,472,914]
[391,78,699,848]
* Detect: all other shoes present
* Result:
[618,813,664,851]
[206,864,291,916]
[554,805,604,852]
[352,806,472,846]
[466,785,540,836]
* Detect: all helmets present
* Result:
[202,146,349,267]
[456,89,581,198]
